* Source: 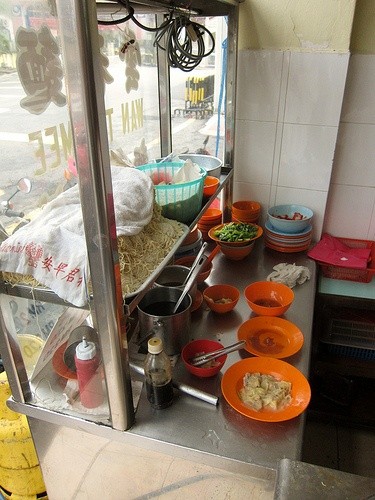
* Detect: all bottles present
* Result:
[74,337,174,408]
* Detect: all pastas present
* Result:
[2,200,184,305]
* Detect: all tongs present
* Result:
[191,340,246,366]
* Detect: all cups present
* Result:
[153,265,197,302]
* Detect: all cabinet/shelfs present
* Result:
[304,294,375,431]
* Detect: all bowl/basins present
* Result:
[181,223,198,245]
[197,209,222,239]
[174,255,213,284]
[182,340,227,378]
[203,284,239,313]
[231,200,314,232]
[216,241,254,260]
[244,281,295,317]
[153,154,222,179]
[203,176,219,196]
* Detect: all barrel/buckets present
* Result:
[137,287,192,355]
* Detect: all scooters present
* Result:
[0,177,32,245]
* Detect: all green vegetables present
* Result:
[213,222,257,241]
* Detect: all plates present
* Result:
[53,341,78,380]
[176,229,202,252]
[221,317,311,422]
[264,221,312,252]
[191,290,203,313]
[208,222,263,246]
